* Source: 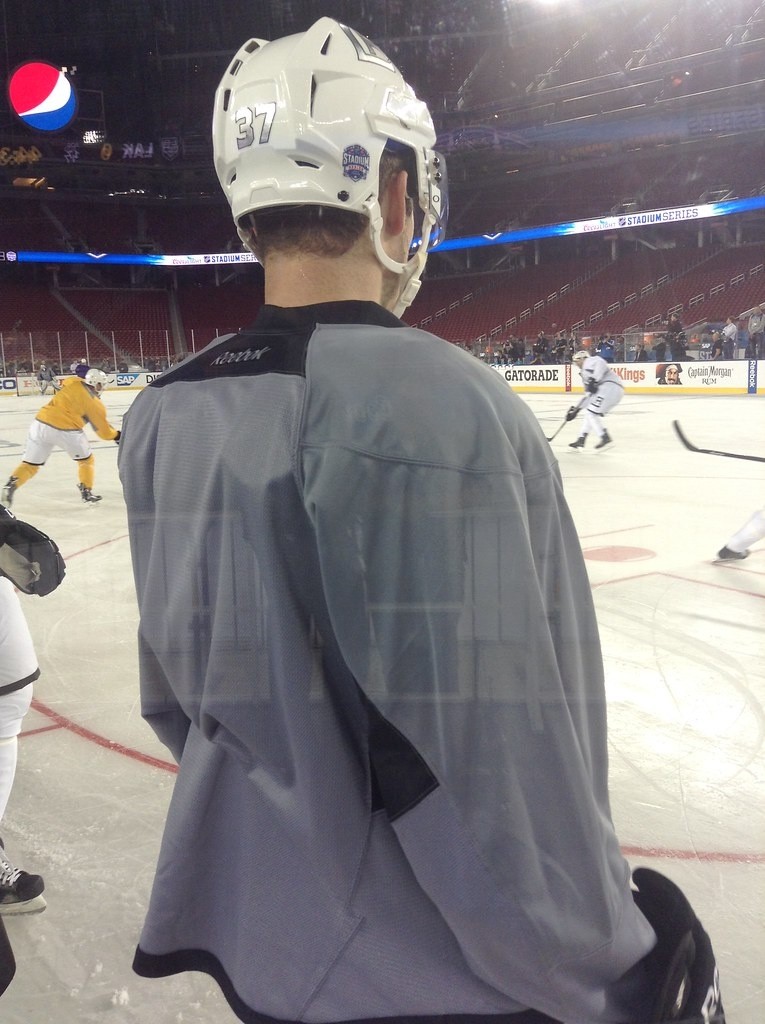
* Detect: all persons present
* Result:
[76,358,90,379]
[665,313,686,361]
[566,350,624,453]
[712,304,765,360]
[651,337,666,362]
[118,358,128,373]
[633,343,648,361]
[494,333,525,364]
[595,332,625,362]
[658,364,682,385]
[2,369,121,508]
[37,364,60,394]
[0,504,66,916]
[530,325,576,364]
[119,15,727,1024]
[100,356,110,374]
[69,359,80,375]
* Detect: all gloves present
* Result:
[566,407,582,421]
[1,505,66,597]
[585,383,598,394]
[612,867,727,1024]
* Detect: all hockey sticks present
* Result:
[544,392,589,442]
[672,418,764,466]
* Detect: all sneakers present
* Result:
[592,428,617,454]
[1,475,20,508]
[711,546,752,565]
[77,483,103,508]
[0,844,48,916]
[566,432,589,453]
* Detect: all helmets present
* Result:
[85,369,108,399]
[211,16,449,320]
[571,350,590,362]
